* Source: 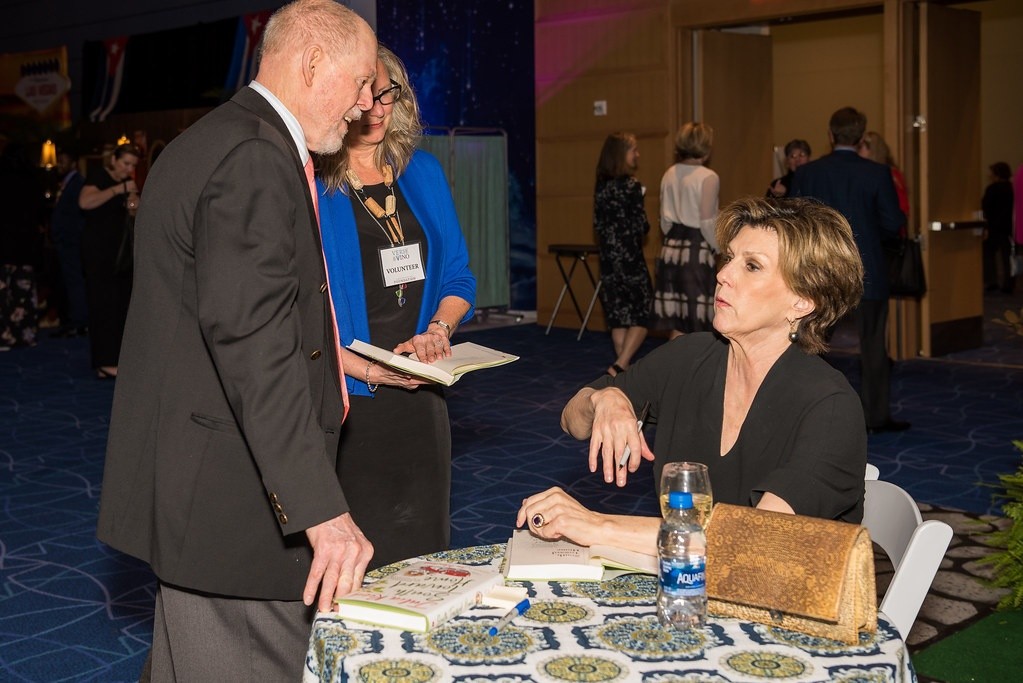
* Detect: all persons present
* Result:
[651,122,721,337]
[790,106,927,434]
[94,1,379,683]
[859,131,910,232]
[0,144,141,377]
[768,139,811,201]
[516,197,869,558]
[592,132,653,377]
[981,162,1023,326]
[315,43,480,565]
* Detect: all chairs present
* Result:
[860,463,954,643]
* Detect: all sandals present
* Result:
[604,363,625,378]
[97,367,117,379]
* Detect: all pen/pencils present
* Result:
[617,399,651,472]
[489,599,531,636]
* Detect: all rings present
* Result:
[130,203,134,206]
[532,513,544,527]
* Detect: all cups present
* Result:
[660,461,713,531]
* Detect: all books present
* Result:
[333,561,504,633]
[500,529,658,582]
[345,338,520,387]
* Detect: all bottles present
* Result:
[656,492,708,632]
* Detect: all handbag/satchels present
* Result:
[881,218,927,302]
[702,502,877,646]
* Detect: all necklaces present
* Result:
[346,169,407,307]
[366,360,378,392]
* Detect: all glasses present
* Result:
[790,152,807,158]
[373,77,401,108]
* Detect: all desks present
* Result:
[302,544,920,683]
[544,244,607,341]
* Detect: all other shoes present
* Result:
[49,328,79,338]
[80,326,89,334]
[863,418,911,434]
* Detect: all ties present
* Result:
[303,154,350,426]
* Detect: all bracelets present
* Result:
[429,320,450,339]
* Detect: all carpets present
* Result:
[910,605,1023,683]
